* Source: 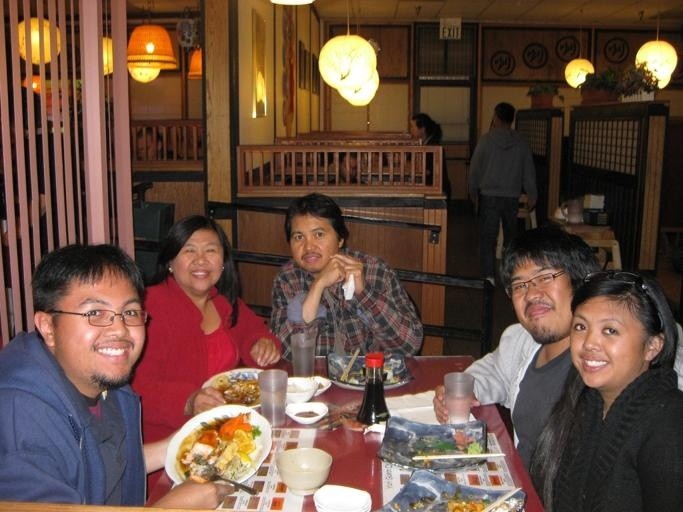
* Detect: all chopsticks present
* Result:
[413,453,506,460]
[481,487,522,512]
[341,349,360,381]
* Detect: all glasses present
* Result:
[583,271,663,332]
[504,271,564,298]
[48,309,148,327]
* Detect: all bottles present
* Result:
[356,353,393,424]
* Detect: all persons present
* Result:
[285,177,311,186]
[136,127,182,160]
[325,154,368,186]
[529,270,683,510]
[467,101,538,287]
[271,193,425,362]
[406,113,451,221]
[0,243,239,511]
[3,89,58,251]
[126,214,288,501]
[433,227,683,473]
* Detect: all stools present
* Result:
[496,193,537,260]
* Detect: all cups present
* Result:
[291,333,317,378]
[444,372,475,425]
[257,369,287,427]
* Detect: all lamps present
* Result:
[17,17,61,65]
[127,17,178,68]
[187,46,204,80]
[126,20,161,83]
[635,20,677,90]
[564,27,595,89]
[318,13,381,106]
[102,36,113,76]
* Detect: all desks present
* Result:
[548,214,623,271]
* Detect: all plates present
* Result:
[373,468,526,512]
[164,405,272,489]
[201,367,264,409]
[326,349,411,392]
[376,416,488,472]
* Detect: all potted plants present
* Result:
[527,82,560,109]
[618,61,661,105]
[577,68,621,106]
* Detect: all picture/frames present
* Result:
[327,24,410,85]
[481,27,594,83]
[298,40,320,96]
[274,6,298,140]
[594,27,683,90]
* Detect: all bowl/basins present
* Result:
[285,402,328,424]
[287,377,317,403]
[276,447,333,497]
[311,376,332,397]
